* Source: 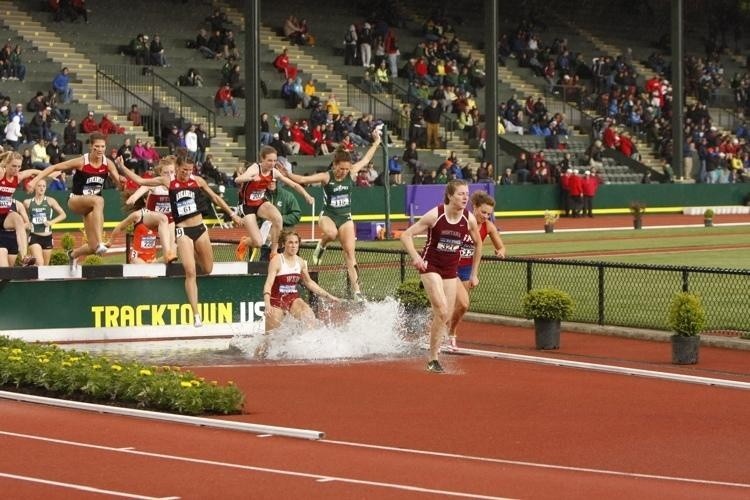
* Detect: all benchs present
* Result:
[0,0,749,185]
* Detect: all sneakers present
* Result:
[352,292,367,305]
[67,249,78,277]
[314,241,326,266]
[164,252,179,264]
[192,312,202,328]
[235,237,249,261]
[18,257,36,267]
[426,358,445,373]
[94,244,107,254]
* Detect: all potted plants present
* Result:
[668,288,707,365]
[394,277,433,334]
[703,207,715,227]
[628,201,647,230]
[519,285,579,351]
[544,209,560,233]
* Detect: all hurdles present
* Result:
[0,260,319,342]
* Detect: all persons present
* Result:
[255,231,340,360]
[444,191,506,352]
[401,180,482,372]
[0,1,749,330]
